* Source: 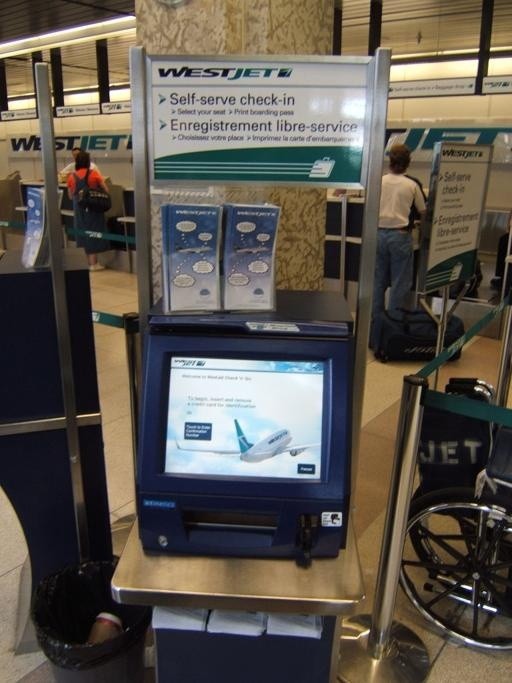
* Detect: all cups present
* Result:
[86,612,122,645]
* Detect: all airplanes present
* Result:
[175,243,214,256]
[175,418,321,465]
[233,243,272,256]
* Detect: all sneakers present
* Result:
[89,262,105,272]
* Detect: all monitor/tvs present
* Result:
[136,334,351,501]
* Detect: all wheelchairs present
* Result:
[398,376,512,653]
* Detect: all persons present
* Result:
[57,146,101,181]
[65,150,113,271]
[390,168,427,249]
[368,142,429,316]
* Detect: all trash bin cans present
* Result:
[30,553,150,682]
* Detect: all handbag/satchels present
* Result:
[77,185,111,212]
[369,307,463,362]
